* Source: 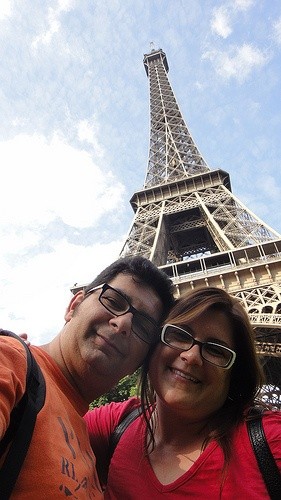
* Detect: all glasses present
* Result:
[160,323,237,370]
[85,283,160,345]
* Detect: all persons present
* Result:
[0,254,176,500]
[0,286,280,500]
